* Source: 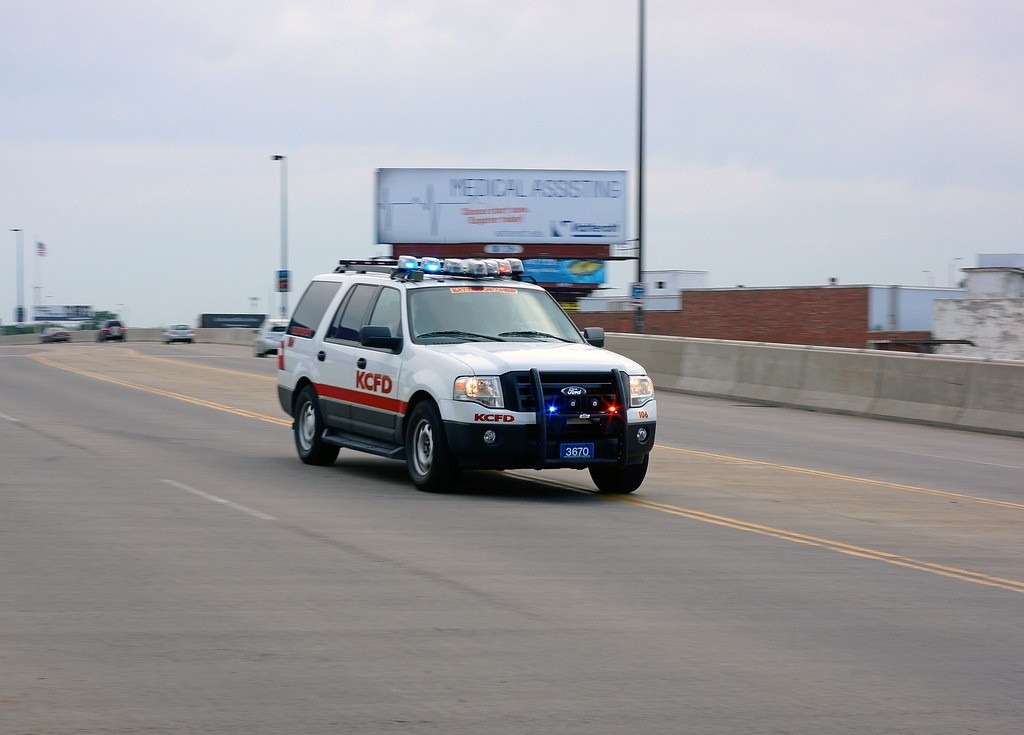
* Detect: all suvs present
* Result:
[275,254,659,495]
[96,319,129,344]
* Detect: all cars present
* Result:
[40,327,73,343]
[162,324,195,344]
[253,318,290,358]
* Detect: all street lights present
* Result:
[9,228,25,321]
[269,153,289,318]
